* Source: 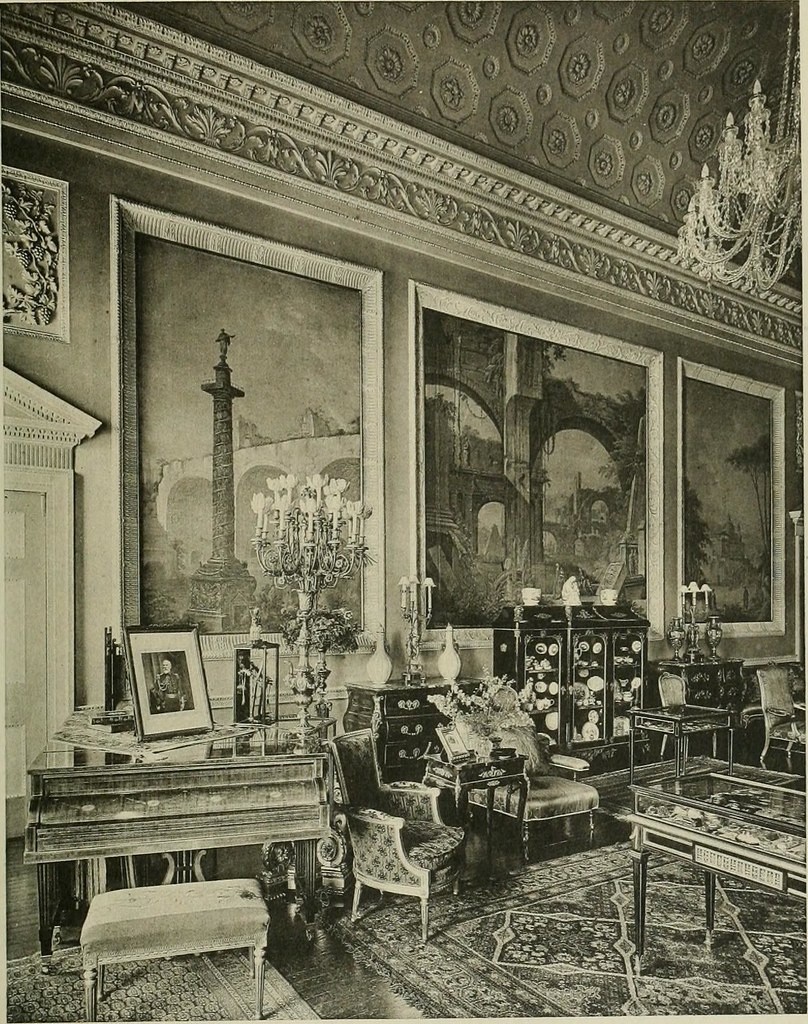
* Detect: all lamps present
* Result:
[398,575,437,686]
[680,582,712,664]
[678,1,801,294]
[252,474,368,730]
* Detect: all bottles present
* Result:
[438,623,461,679]
[367,625,392,684]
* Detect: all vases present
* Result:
[707,616,723,662]
[668,618,686,662]
[475,737,492,758]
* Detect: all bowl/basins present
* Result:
[601,590,617,605]
[522,588,541,604]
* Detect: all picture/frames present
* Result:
[435,725,470,764]
[676,356,786,640]
[1,162,71,344]
[408,278,665,650]
[121,625,214,744]
[108,194,386,661]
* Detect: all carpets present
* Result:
[578,756,804,817]
[7,945,323,1022]
[328,840,808,1019]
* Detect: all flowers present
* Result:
[427,664,523,738]
[279,605,361,654]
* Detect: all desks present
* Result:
[627,705,736,785]
[626,773,806,956]
[423,753,531,862]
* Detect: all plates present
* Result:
[526,641,641,748]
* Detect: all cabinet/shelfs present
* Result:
[343,677,484,782]
[655,658,747,712]
[492,605,652,763]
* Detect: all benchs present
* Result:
[79,878,271,1021]
[740,662,805,730]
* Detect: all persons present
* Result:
[154,659,184,712]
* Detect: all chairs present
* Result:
[657,672,687,755]
[455,709,600,859]
[755,663,806,762]
[329,728,465,940]
